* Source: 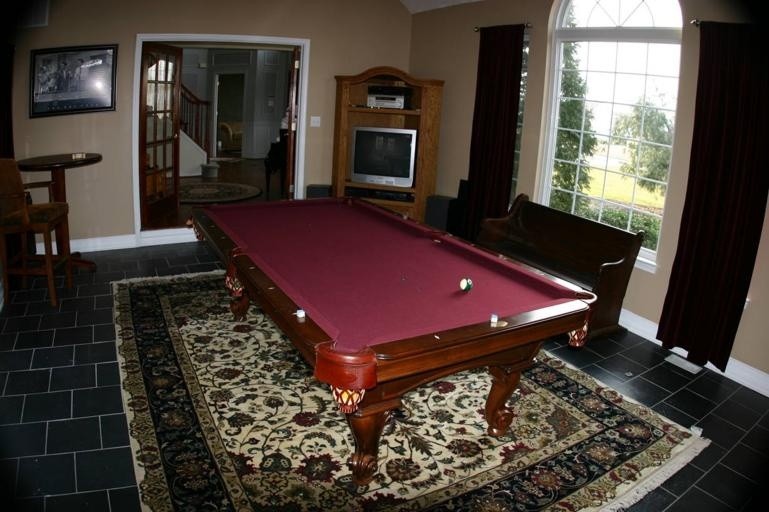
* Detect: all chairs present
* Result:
[0,158,73,307]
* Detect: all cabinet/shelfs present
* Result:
[331,66,445,222]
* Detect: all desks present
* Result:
[188,196,598,486]
[16,153,103,274]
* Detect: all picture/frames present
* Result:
[29,43,118,119]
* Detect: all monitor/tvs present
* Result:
[348,126,417,188]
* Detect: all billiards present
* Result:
[459,278,475,291]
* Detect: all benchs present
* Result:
[479,193,644,338]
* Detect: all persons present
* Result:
[35,57,104,96]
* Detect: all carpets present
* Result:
[160,182,263,205]
[109,269,712,512]
